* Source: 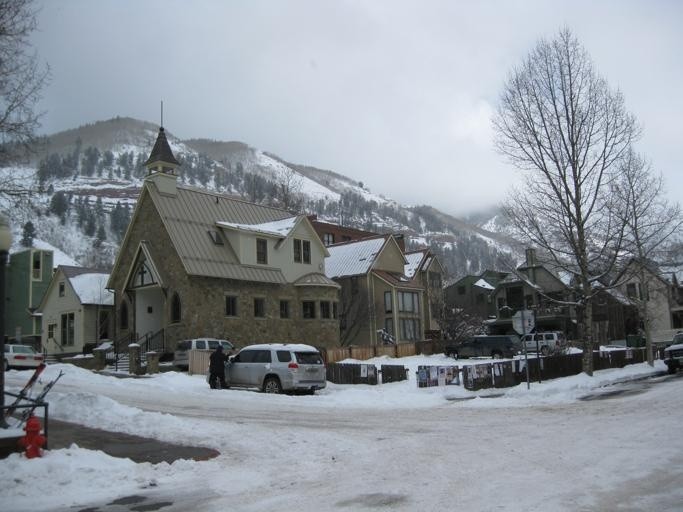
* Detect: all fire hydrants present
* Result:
[20,416,46,459]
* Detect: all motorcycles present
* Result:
[377,328,395,345]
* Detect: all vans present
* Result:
[453,331,567,360]
[174,337,326,394]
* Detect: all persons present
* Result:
[209,345,230,389]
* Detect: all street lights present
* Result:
[0,214,12,429]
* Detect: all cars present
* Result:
[3,344,44,371]
[664,331,683,373]
